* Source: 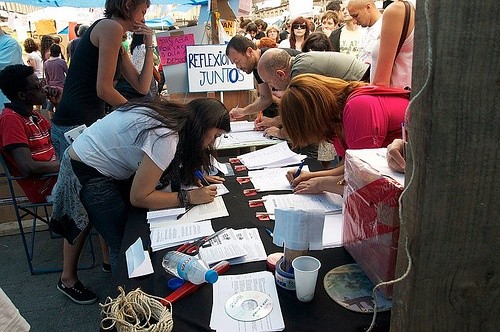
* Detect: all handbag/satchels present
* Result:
[41,80,46,86]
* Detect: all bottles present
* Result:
[162,251,218,285]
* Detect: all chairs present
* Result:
[0,153,95,276]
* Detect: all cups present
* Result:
[292,255,321,302]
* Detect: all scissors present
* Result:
[177,227,228,256]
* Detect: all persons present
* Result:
[279,73,410,195]
[0,0,417,305]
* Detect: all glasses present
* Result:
[293,24,307,29]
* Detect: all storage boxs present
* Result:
[339,148,407,298]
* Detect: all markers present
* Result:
[194,170,210,187]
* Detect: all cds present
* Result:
[224,291,273,321]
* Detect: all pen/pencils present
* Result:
[288,158,307,188]
[254,111,263,132]
[265,227,272,235]
[401,121,407,160]
[175,204,195,220]
[231,104,240,117]
[224,134,228,137]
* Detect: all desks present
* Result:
[210,120,287,153]
[103,157,392,332]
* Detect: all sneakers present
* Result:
[57,276,99,304]
[101,261,113,272]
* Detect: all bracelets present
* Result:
[145,44,154,51]
[177,189,188,207]
